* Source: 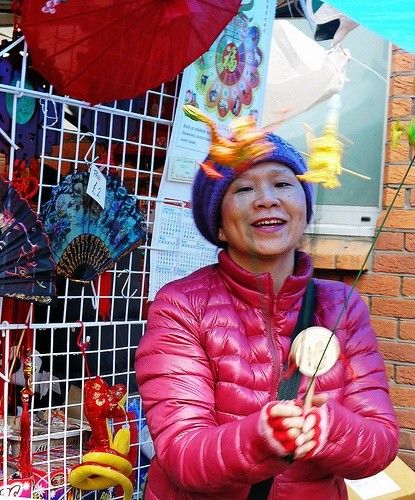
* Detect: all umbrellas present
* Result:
[19,0,241,107]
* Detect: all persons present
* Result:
[134,108,400,500]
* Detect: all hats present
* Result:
[192,134,313,249]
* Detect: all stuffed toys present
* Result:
[68,377,134,500]
